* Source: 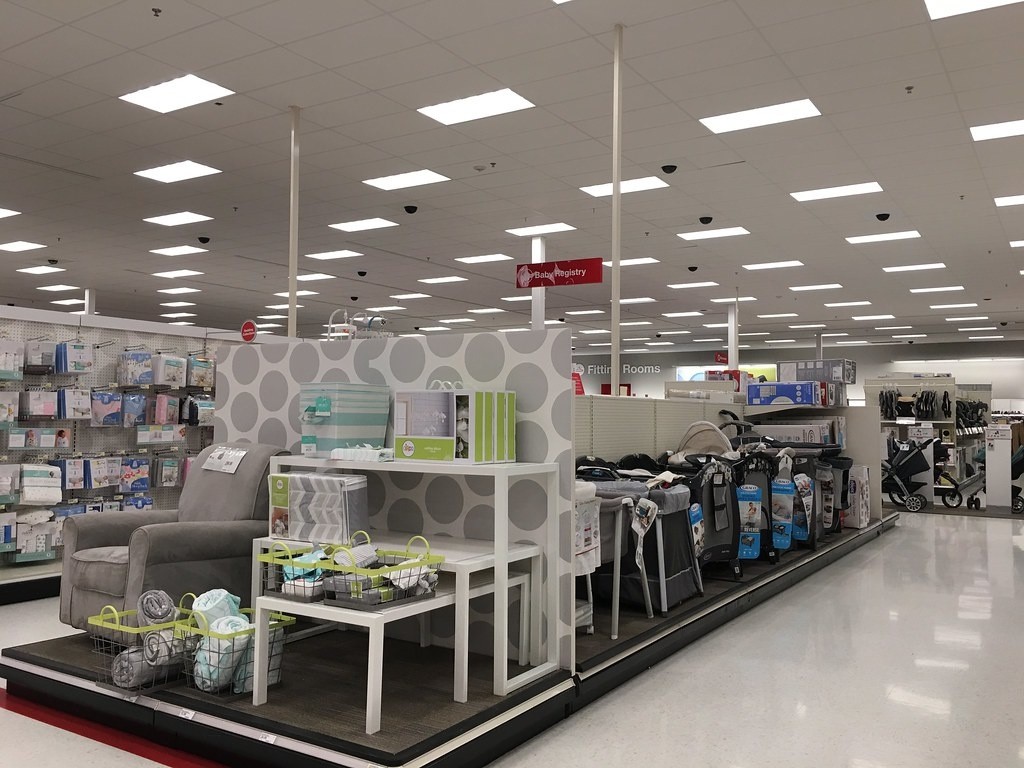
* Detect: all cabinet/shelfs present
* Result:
[861,377,1024,514]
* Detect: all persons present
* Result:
[73,406,90,415]
[66,473,119,487]
[171,428,186,441]
[27,430,38,447]
[56,430,69,447]
[95,413,102,425]
[125,413,135,425]
[745,502,756,529]
[71,359,94,371]
[694,520,706,552]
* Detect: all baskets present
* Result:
[316,535,446,614]
[88,592,199,698]
[173,605,296,704]
[256,530,371,602]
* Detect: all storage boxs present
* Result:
[842,384,848,406]
[268,471,371,546]
[842,417,848,451]
[941,471,951,486]
[494,392,506,465]
[820,382,829,406]
[298,381,390,393]
[828,384,836,405]
[777,414,844,448]
[393,389,483,465]
[941,428,952,442]
[484,390,493,464]
[963,438,979,472]
[298,406,390,457]
[839,475,867,530]
[751,425,824,444]
[776,359,852,383]
[0,339,215,565]
[933,428,939,439]
[956,449,963,482]
[847,361,856,385]
[300,390,390,402]
[506,390,518,463]
[299,398,390,407]
[961,445,976,474]
[747,381,823,405]
[703,370,748,394]
[957,447,966,480]
[832,381,843,406]
[938,448,955,463]
[760,419,836,444]
[850,465,872,523]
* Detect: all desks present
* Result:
[252,571,530,732]
[247,529,544,698]
[269,454,562,692]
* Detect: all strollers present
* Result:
[966,444,1024,513]
[932,436,963,509]
[881,429,934,512]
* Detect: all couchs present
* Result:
[61,442,291,651]
